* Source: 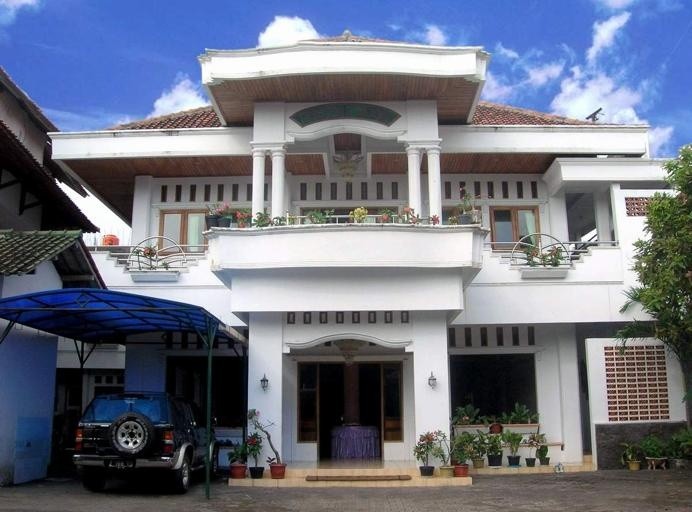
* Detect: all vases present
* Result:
[249,466,264,478]
[269,461,288,480]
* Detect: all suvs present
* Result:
[73,392,218,494]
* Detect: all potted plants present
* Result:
[412,401,554,478]
[226,443,247,478]
[618,426,692,471]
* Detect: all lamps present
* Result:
[334,159,360,181]
[428,370,439,391]
[260,373,270,393]
[333,338,367,369]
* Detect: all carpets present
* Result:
[305,473,414,484]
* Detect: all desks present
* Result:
[331,422,383,461]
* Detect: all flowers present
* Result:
[245,431,265,467]
[204,183,497,233]
[245,405,283,467]
